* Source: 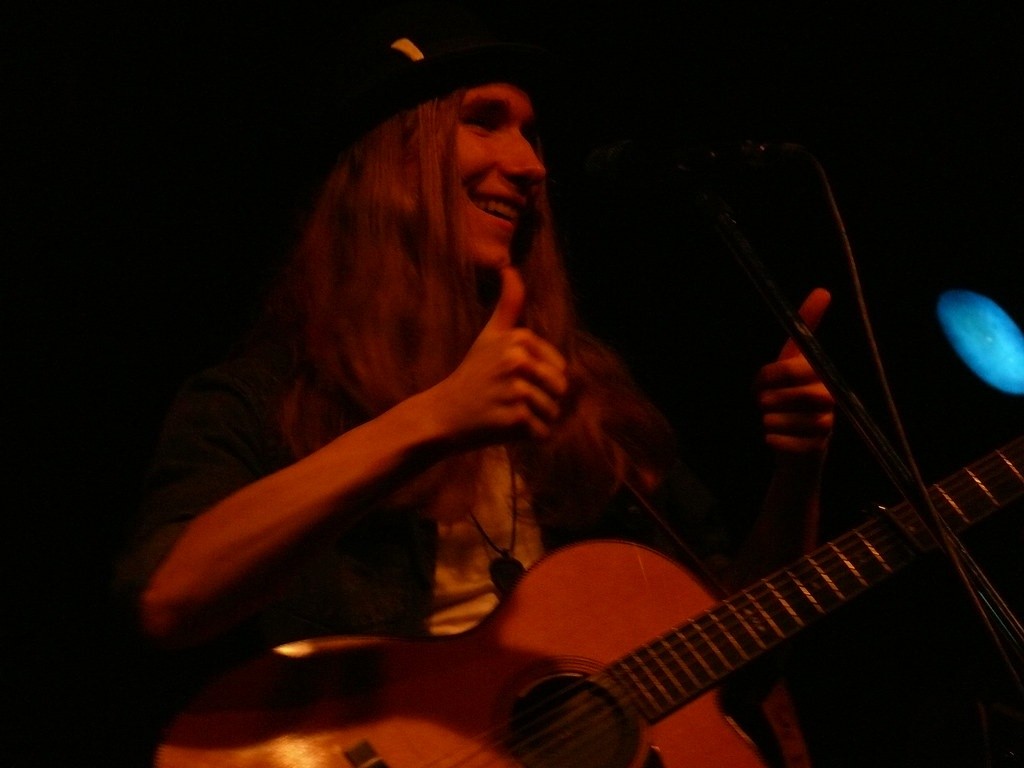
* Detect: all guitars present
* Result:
[152,436,1024,768]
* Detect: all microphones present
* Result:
[583,133,804,183]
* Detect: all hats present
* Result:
[300,34,539,184]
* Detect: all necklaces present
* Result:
[464,445,526,599]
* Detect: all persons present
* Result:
[125,33,834,768]
